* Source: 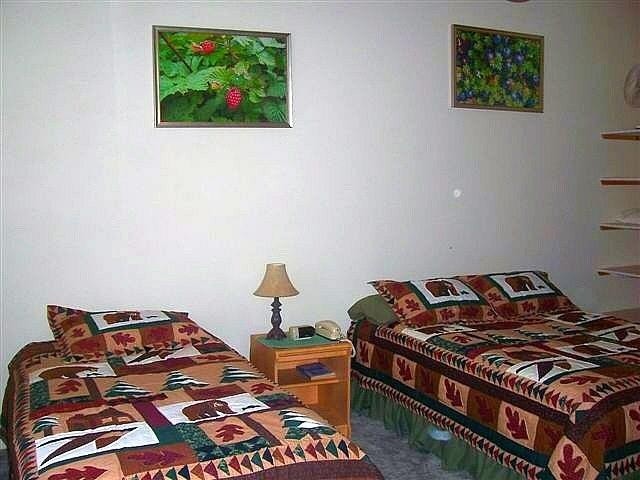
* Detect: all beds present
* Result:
[1,330,386,480]
[347,308,640,480]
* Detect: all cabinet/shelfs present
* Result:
[598,128,640,280]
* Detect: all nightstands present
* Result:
[248,330,353,445]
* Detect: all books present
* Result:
[295,362,336,381]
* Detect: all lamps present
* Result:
[252,262,302,340]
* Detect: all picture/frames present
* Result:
[150,23,294,129]
[450,23,546,114]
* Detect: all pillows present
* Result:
[349,296,395,326]
[456,268,589,318]
[46,302,208,359]
[369,275,497,330]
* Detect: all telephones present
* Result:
[315,320,341,340]
